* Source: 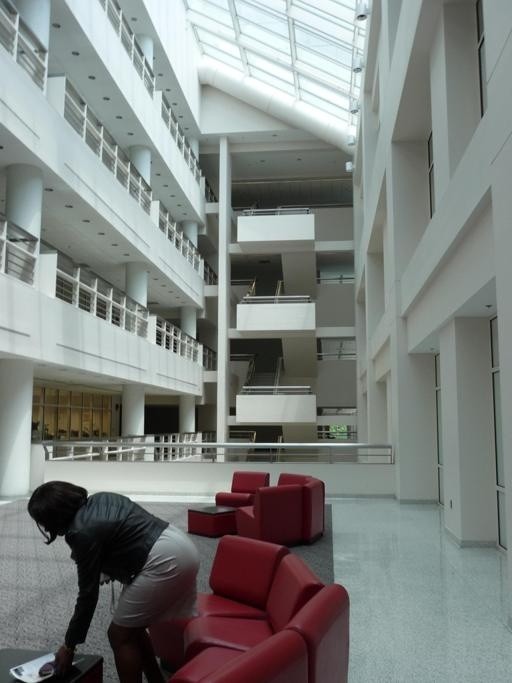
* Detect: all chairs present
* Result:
[146,534,290,674]
[214,471,325,547]
[185,553,325,664]
[280,584,350,683]
[169,629,310,682]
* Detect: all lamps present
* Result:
[352,57,362,73]
[347,135,355,145]
[346,163,353,173]
[356,3,368,21]
[350,101,359,114]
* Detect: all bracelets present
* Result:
[62,642,79,653]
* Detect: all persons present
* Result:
[26,484,200,682]
